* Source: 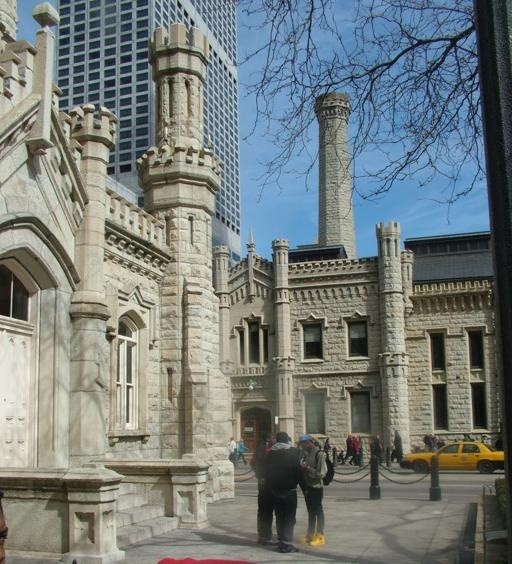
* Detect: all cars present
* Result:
[401,442,505,474]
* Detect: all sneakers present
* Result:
[276,543,298,553]
[258,535,280,544]
[310,534,325,546]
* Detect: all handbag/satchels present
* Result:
[316,449,334,485]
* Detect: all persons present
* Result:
[248,430,276,548]
[265,431,302,553]
[297,433,329,546]
[228,429,491,469]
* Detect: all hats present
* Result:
[276,432,288,442]
[299,434,314,443]
